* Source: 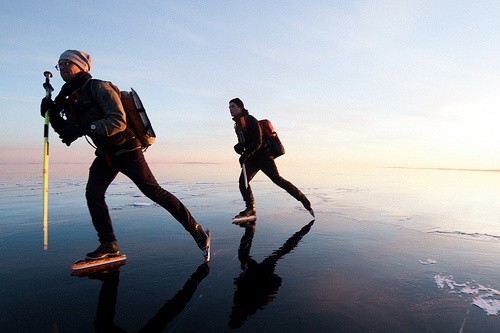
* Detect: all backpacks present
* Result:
[81,77,156,152]
[240,115,285,159]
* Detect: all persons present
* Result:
[40,49,211,263]
[229,98,315,223]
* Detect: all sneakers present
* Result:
[192,223,210,251]
[86,239,120,260]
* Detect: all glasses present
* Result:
[55,62,70,71]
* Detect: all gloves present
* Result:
[40,97,56,117]
[234,143,245,155]
[58,124,83,143]
[239,149,251,168]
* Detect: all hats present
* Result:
[58,50,90,72]
[229,98,244,109]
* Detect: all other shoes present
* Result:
[239,204,256,216]
[301,197,311,211]
